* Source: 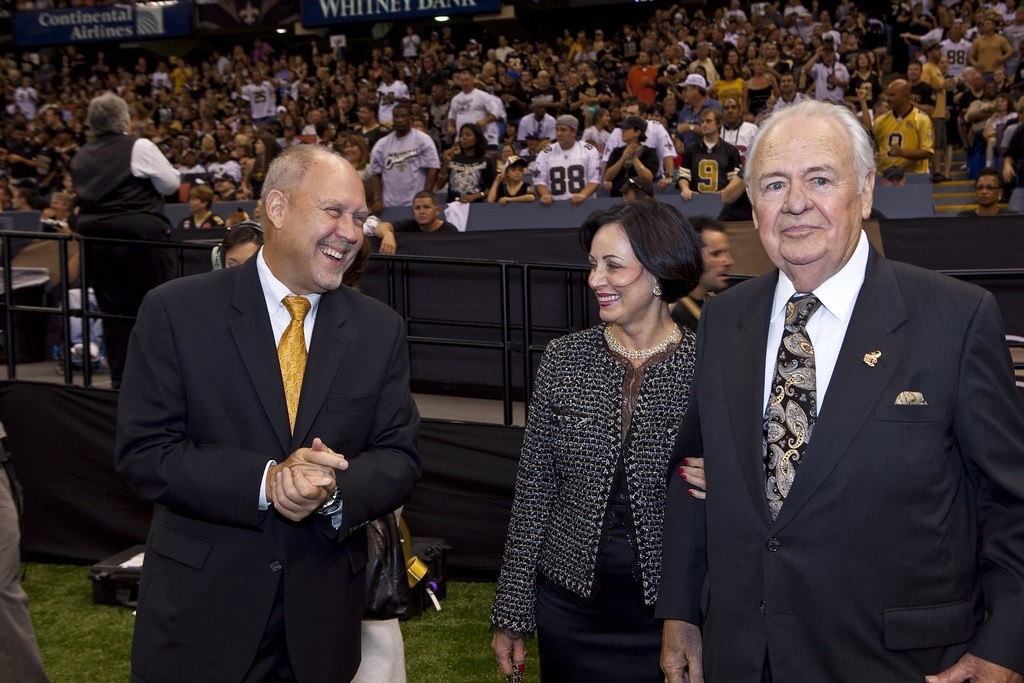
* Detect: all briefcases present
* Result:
[407,535,449,613]
[90,544,148,608]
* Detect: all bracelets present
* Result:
[315,482,342,515]
[480,191,485,197]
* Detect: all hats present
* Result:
[618,116,647,132]
[679,74,706,88]
[555,115,577,129]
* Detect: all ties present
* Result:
[277,296,310,437]
[760,293,820,522]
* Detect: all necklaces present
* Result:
[603,322,681,359]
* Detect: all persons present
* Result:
[436,122,493,203]
[486,154,536,206]
[68,90,180,389]
[667,214,735,334]
[113,144,424,683]
[368,103,441,214]
[446,70,501,156]
[374,190,459,257]
[532,114,602,207]
[0,0,1024,367]
[487,196,706,683]
[603,116,660,198]
[655,92,1024,683]
[855,78,936,175]
[674,108,744,201]
[210,220,264,271]
[957,168,1022,217]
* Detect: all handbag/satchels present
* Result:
[345,512,415,620]
